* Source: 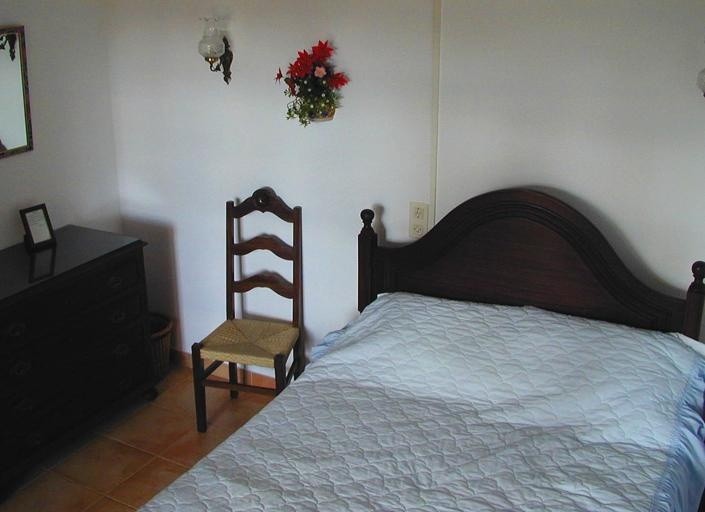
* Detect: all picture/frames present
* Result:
[18,203,57,251]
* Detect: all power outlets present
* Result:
[410,202,428,239]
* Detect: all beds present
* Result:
[136,188,704,511]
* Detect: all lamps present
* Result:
[195,13,233,84]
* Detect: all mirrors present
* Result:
[0,26,32,158]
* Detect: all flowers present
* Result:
[274,40,349,126]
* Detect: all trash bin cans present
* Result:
[137,312,173,374]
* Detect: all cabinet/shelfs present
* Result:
[0,225,160,502]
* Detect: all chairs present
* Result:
[192,187,302,434]
